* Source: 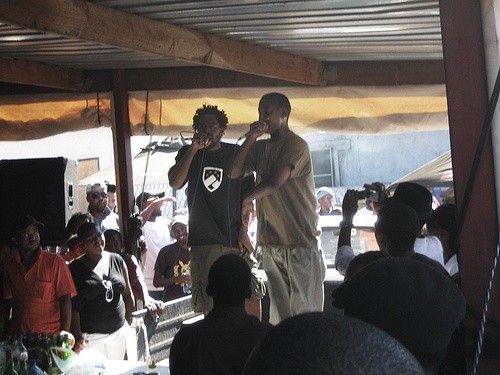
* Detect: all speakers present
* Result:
[0,156,81,247]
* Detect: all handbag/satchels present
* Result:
[50,346,129,375]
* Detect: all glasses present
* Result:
[90,192,107,200]
[102,280,114,303]
[18,229,39,239]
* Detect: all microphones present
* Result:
[238,126,260,140]
[185,135,209,144]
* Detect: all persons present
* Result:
[103,229,157,316]
[312,178,460,288]
[133,285,165,312]
[335,181,447,270]
[169,252,274,375]
[55,182,260,254]
[152,215,193,300]
[241,312,426,375]
[168,104,255,317]
[0,215,78,375]
[68,222,135,355]
[327,202,467,375]
[226,93,326,322]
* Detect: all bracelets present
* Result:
[338,222,354,228]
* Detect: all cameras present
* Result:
[358,189,382,202]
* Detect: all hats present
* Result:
[384,182,436,221]
[168,215,188,237]
[136,192,165,207]
[316,187,333,201]
[6,213,45,239]
[86,180,107,193]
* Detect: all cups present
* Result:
[41,246,69,254]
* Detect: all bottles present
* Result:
[183,272,192,294]
[147,361,160,375]
[0,329,73,375]
